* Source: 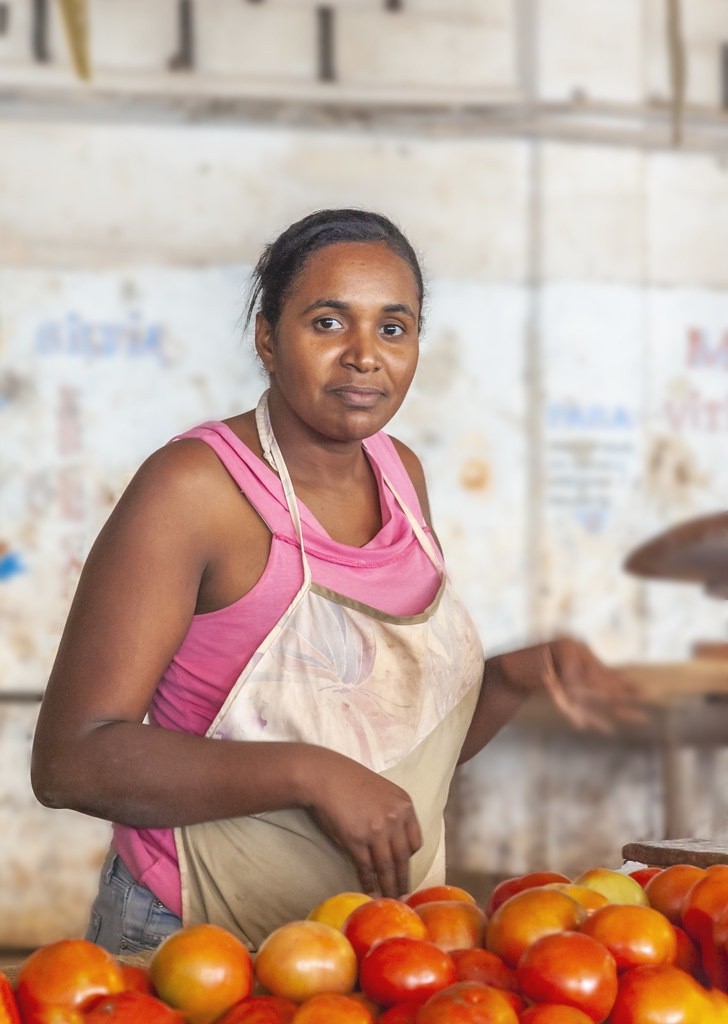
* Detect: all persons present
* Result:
[32,209,634,958]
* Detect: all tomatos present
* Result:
[0,863,728,1024]
